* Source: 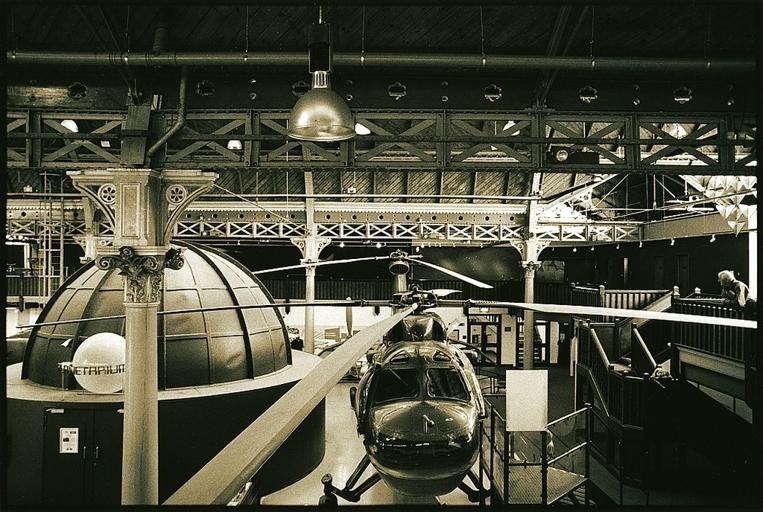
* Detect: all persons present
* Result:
[290,334,304,351]
[717,269,757,308]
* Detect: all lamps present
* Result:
[285,6,357,144]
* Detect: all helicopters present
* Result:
[10,248,757,506]
